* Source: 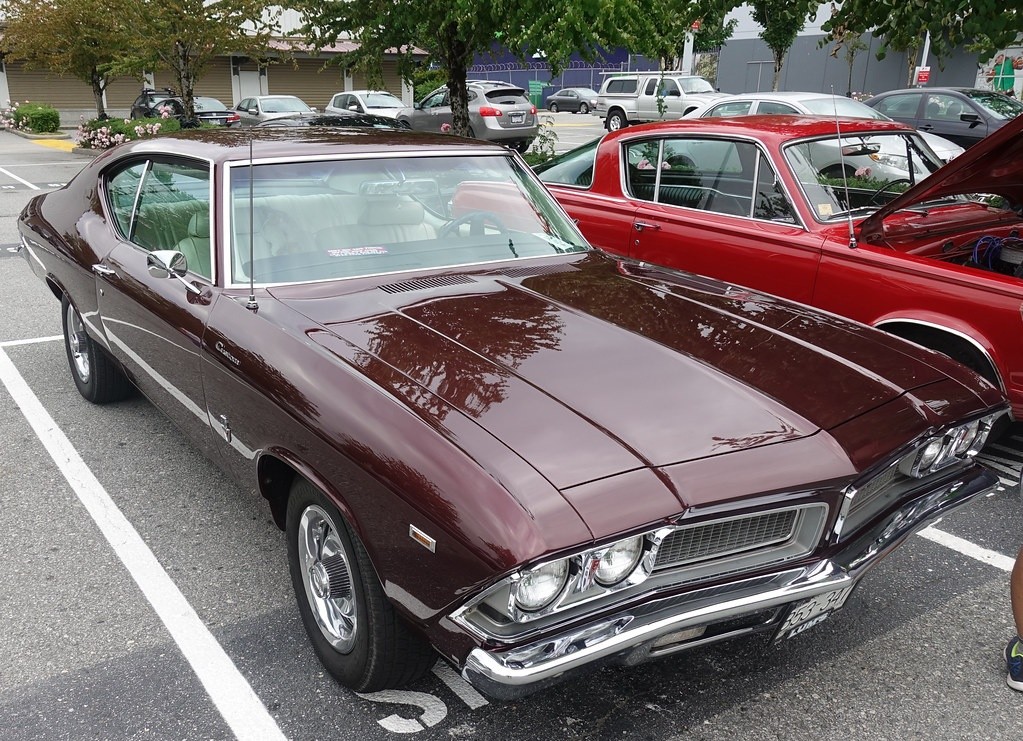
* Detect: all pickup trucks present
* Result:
[592,68,739,133]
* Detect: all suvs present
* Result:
[395,79,540,154]
[130,87,177,122]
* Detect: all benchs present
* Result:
[123,193,361,257]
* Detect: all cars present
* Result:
[859,85,1023,154]
[229,94,318,128]
[251,110,415,131]
[324,89,409,121]
[12,119,1018,707]
[544,87,599,114]
[143,95,242,129]
[651,90,968,195]
[448,111,1023,455]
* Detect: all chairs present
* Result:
[357,199,436,245]
[926,103,940,119]
[172,210,270,282]
[946,103,961,118]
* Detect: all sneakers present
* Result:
[1004,635,1023,691]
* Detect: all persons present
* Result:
[986,50,1022,100]
[1002,547,1023,691]
